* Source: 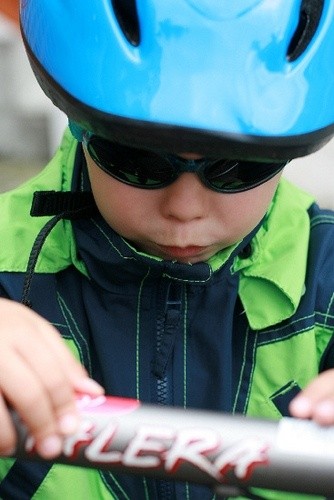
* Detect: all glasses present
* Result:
[68,122,290,193]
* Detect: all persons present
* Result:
[0,0,334,500]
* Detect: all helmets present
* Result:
[18,0,333,159]
